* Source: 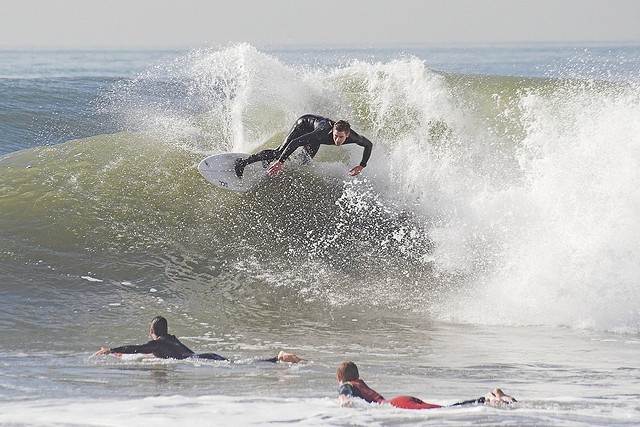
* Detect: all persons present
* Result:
[336,361,514,408]
[94,316,305,366]
[235,114,373,180]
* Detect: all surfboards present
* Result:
[198,151,266,192]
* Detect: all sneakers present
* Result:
[236,157,246,178]
